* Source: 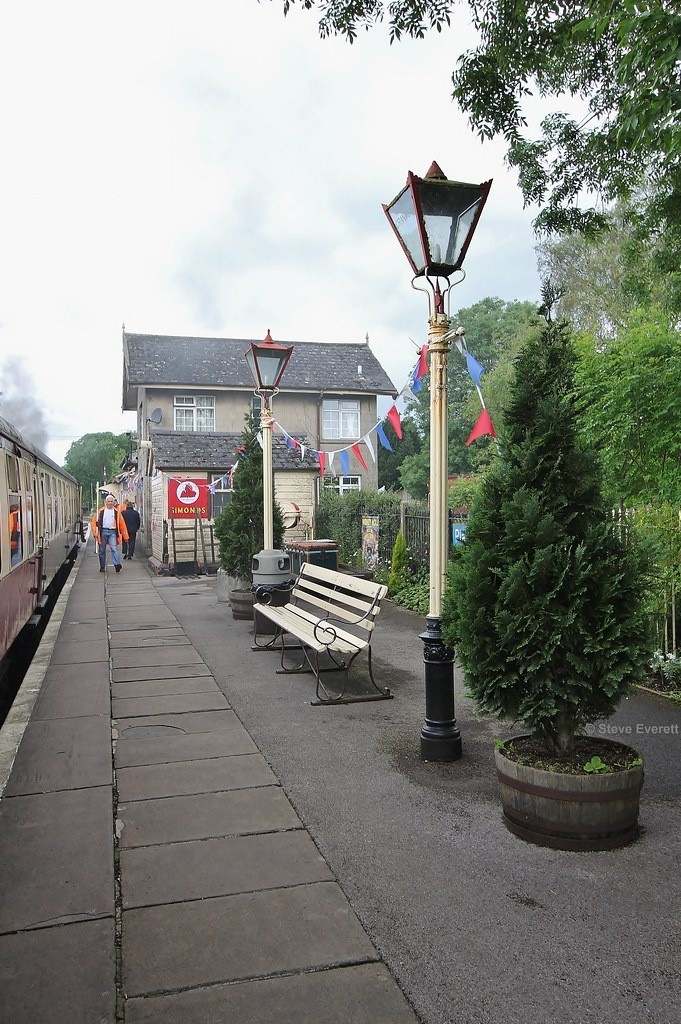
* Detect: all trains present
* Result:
[1,420,82,663]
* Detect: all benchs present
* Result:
[250,562,394,706]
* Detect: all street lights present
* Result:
[246,330,297,640]
[384,161,490,763]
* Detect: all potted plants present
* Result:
[437,273,669,850]
[213,402,287,620]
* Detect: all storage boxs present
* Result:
[286,541,339,577]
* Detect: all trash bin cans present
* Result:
[251,549,292,635]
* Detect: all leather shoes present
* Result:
[99,567,106,573]
[115,564,122,573]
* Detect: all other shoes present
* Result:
[123,555,133,560]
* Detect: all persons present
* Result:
[92,495,129,572]
[121,502,140,560]
[120,499,129,513]
[9,498,34,567]
